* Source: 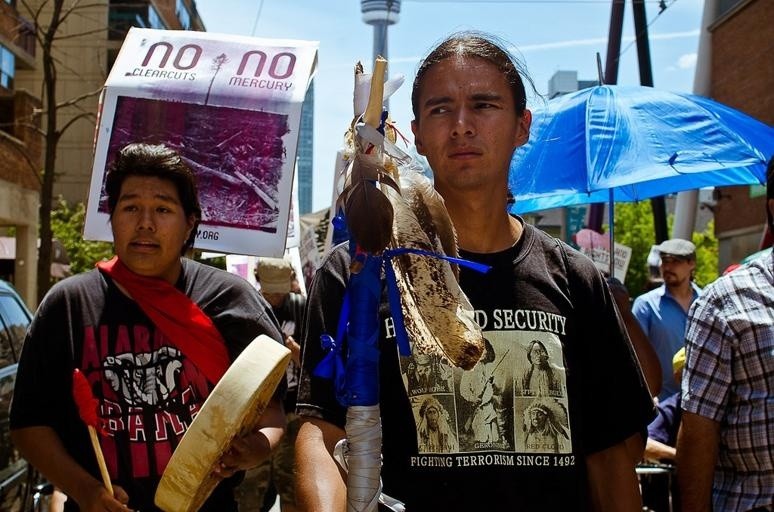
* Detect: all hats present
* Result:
[673,347,687,372]
[657,238,697,257]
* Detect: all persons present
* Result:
[634,239,743,512]
[0,139,299,509]
[255,236,364,512]
[670,155,773,512]
[273,26,661,512]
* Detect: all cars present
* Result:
[1,279,55,511]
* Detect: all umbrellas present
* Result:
[500,81,774,277]
